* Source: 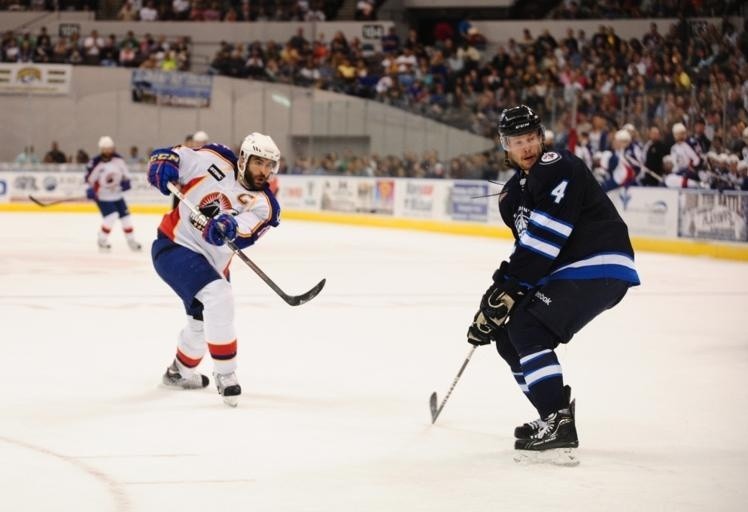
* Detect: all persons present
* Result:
[84,136,141,248]
[189,2,748,134]
[541,114,748,191]
[147,133,283,393]
[15,133,210,163]
[279,147,518,183]
[0,1,189,72]
[465,102,642,452]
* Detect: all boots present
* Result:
[208,340,243,397]
[120,226,143,250]
[514,398,580,451]
[95,229,112,246]
[161,341,209,390]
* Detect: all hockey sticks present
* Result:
[29,196,83,206]
[166,180,325,306]
[430,345,478,423]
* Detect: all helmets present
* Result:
[98,135,116,155]
[237,131,282,183]
[193,130,209,154]
[496,104,546,162]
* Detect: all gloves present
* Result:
[464,261,535,346]
[120,180,132,191]
[85,186,97,199]
[146,148,181,195]
[199,211,242,250]
